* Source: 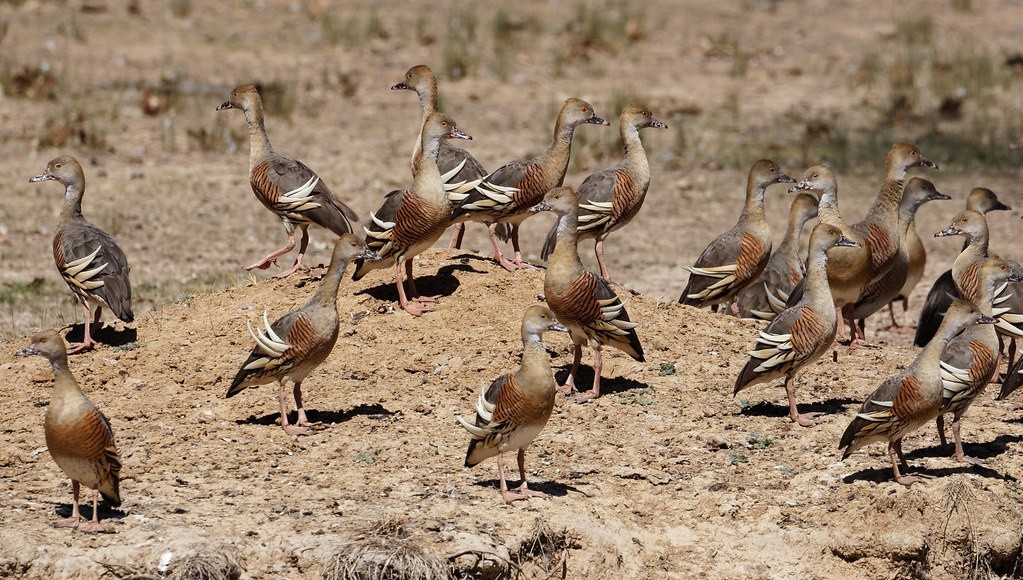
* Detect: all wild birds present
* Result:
[29,155,135,356]
[226,233,386,437]
[14,329,123,532]
[526,186,648,405]
[351,64,1023,383]
[837,299,1000,488]
[732,223,863,428]
[456,304,572,506]
[215,84,361,281]
[935,255,1023,465]
